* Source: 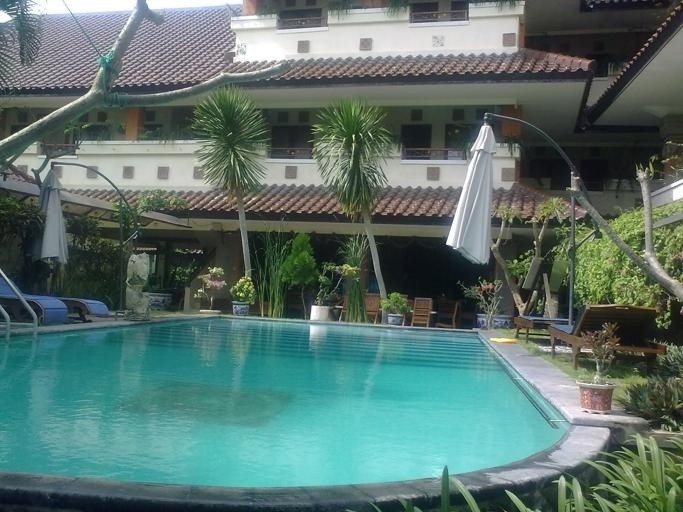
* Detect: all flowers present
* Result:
[197,266,224,309]
[229,276,258,305]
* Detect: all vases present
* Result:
[199,310,221,315]
[473,311,511,329]
[232,300,249,316]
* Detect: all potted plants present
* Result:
[575,320,622,415]
[306,260,357,320]
[144,268,174,312]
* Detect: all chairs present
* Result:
[548,302,666,374]
[0,274,68,325]
[332,288,464,328]
[36,295,109,323]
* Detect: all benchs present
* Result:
[511,313,570,343]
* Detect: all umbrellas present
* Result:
[27,166,70,296]
[443,111,501,356]
